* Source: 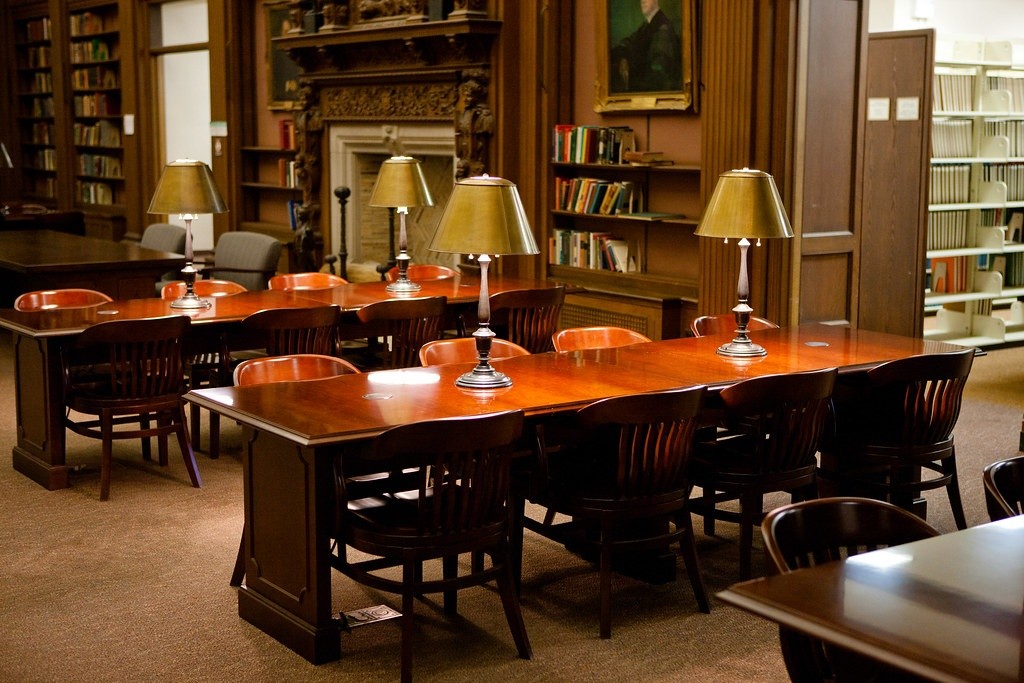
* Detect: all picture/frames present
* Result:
[593,0,698,115]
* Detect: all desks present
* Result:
[181,320,988,666]
[0,229,187,307]
[713,513,1024,683]
[0,272,587,493]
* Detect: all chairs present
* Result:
[13,222,1024,683]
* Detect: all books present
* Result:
[551,124,676,167]
[549,229,639,275]
[278,119,303,232]
[554,175,686,222]
[920,71,1024,316]
[29,10,123,205]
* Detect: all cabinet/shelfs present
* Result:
[923,63,1024,356]
[547,160,702,302]
[5,0,152,244]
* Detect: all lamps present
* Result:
[367,155,434,292]
[427,174,541,391]
[693,167,795,357]
[147,158,231,310]
